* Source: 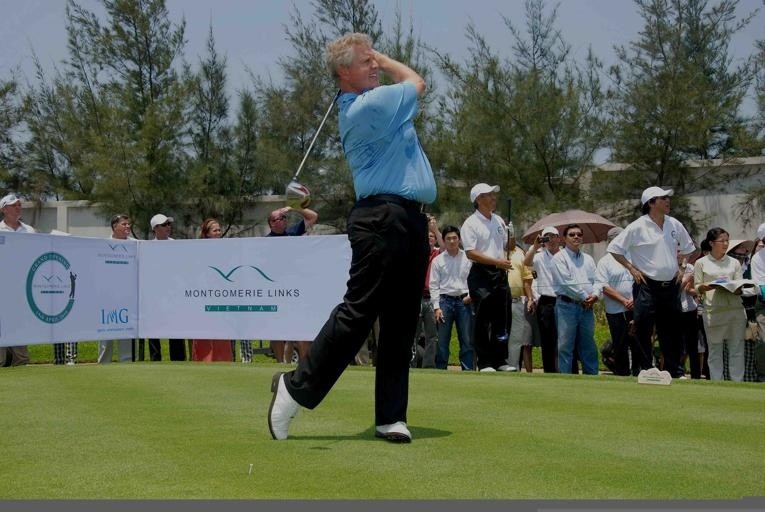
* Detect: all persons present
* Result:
[267,32,438,444]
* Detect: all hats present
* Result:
[540,226,560,238]
[469,181,500,204]
[150,213,175,230]
[1,194,23,209]
[637,184,676,207]
[725,239,756,257]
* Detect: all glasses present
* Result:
[160,222,171,227]
[566,232,583,237]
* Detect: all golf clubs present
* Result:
[288,85,343,207]
[493,197,512,345]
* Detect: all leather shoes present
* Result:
[498,363,518,374]
[478,365,497,375]
[375,419,414,446]
[265,368,301,444]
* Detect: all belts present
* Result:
[371,194,426,216]
[441,292,469,302]
[646,278,676,287]
[511,298,521,303]
[560,294,583,306]
